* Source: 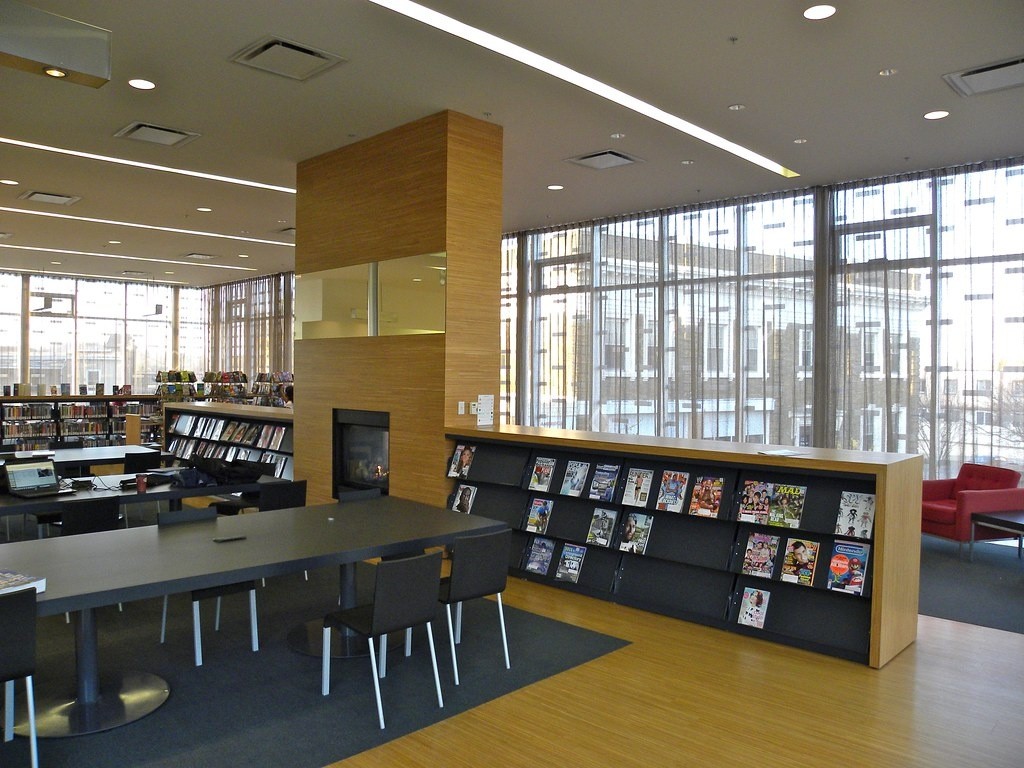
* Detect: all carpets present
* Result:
[0,475,638,768]
[917,530,1024,635]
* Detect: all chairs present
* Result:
[123,451,161,529]
[58,495,123,624]
[0,440,95,487]
[257,480,309,587]
[406,528,512,687]
[321,550,444,730]
[920,463,1024,562]
[149,505,259,667]
[208,462,277,518]
[0,586,40,768]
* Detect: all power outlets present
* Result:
[457,401,464,415]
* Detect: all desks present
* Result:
[0,495,510,740]
[970,509,1024,562]
[0,444,160,478]
[0,466,289,517]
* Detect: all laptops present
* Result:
[4,458,77,499]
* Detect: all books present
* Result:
[768,482,807,529]
[689,476,723,518]
[779,538,819,587]
[738,586,770,629]
[447,445,477,515]
[742,532,779,579]
[525,458,654,584]
[826,490,876,596]
[2,404,162,452]
[655,470,689,514]
[168,415,287,479]
[736,480,773,525]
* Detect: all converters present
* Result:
[72,480,91,488]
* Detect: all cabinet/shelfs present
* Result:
[0,394,172,450]
[445,423,925,669]
[162,402,294,482]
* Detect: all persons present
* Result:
[535,501,549,530]
[456,487,472,511]
[790,541,814,578]
[535,461,546,486]
[740,484,771,521]
[455,447,472,476]
[634,475,643,503]
[661,473,686,501]
[621,514,637,550]
[743,591,764,625]
[744,535,776,577]
[691,478,722,515]
